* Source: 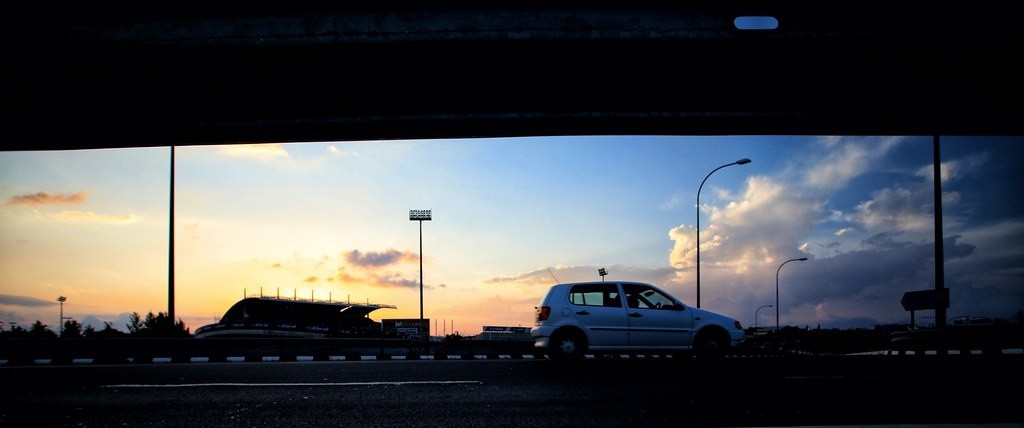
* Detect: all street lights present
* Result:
[696,158,753,308]
[776,257,809,330]
[598,268,608,282]
[57,296,66,335]
[408,208,433,336]
[755,305,774,328]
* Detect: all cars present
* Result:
[530,282,746,360]
